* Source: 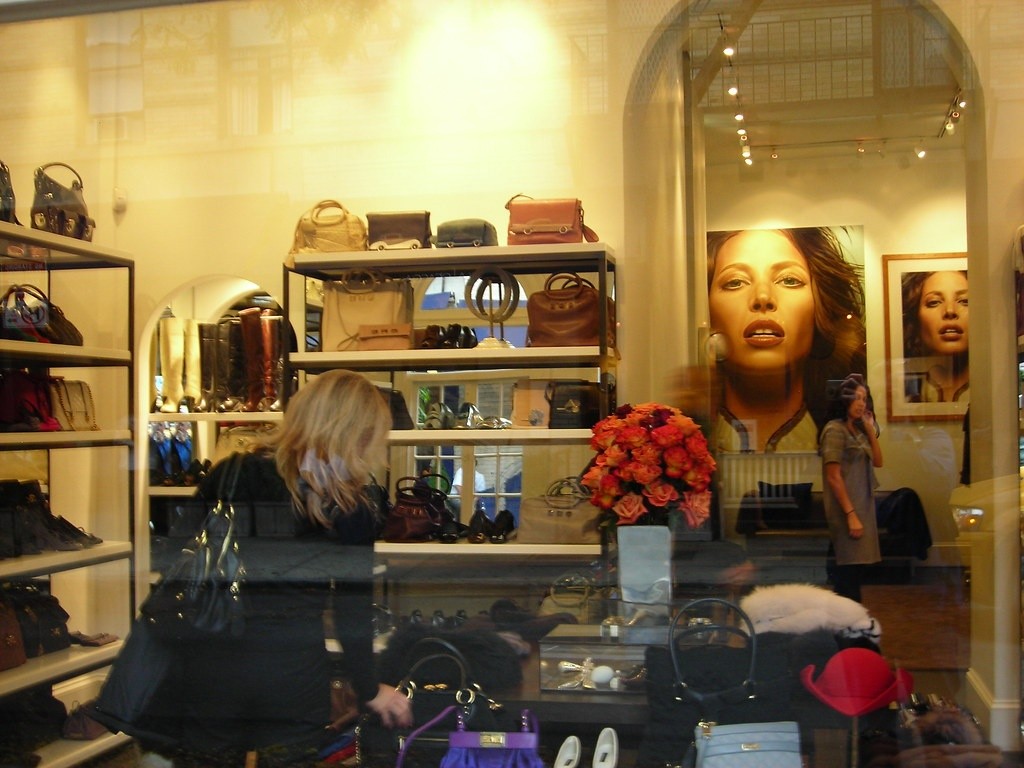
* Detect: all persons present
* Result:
[902,270,971,404]
[707,227,869,452]
[817,377,883,599]
[159,369,413,768]
[448,459,485,511]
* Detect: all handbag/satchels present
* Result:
[509,376,587,426]
[693,718,801,768]
[359,472,390,527]
[341,572,611,768]
[287,193,599,257]
[355,324,415,351]
[372,385,414,430]
[383,474,450,542]
[641,597,782,768]
[321,269,414,352]
[543,378,617,429]
[138,451,249,649]
[0,162,99,436]
[0,575,120,768]
[525,270,615,346]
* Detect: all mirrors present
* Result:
[135,272,297,583]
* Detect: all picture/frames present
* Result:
[882,251,974,421]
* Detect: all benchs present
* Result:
[732,480,924,579]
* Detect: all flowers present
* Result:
[582,403,719,533]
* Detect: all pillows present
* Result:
[757,482,813,529]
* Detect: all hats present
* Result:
[804,648,913,715]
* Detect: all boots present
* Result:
[152,307,286,414]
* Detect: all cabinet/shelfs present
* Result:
[149,239,616,579]
[0,215,146,768]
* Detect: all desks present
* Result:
[753,527,888,539]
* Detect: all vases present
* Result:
[615,524,674,606]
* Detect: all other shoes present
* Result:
[0,478,103,559]
[420,322,509,428]
[434,505,517,545]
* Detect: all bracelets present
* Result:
[846,510,854,515]
